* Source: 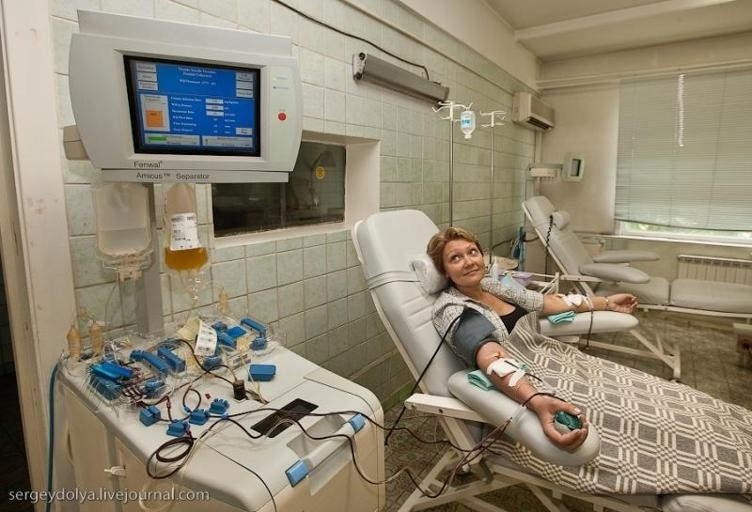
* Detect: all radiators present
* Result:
[675,255,751,286]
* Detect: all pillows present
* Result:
[410,255,449,293]
[550,209,571,229]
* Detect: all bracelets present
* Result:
[603,295,609,311]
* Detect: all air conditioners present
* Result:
[512,92,556,133]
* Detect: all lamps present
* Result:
[352,54,449,107]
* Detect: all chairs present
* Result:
[357,196,752,512]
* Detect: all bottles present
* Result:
[462,111,475,139]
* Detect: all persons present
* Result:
[427,227,638,454]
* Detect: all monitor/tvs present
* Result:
[68,32,304,171]
[563,153,584,181]
